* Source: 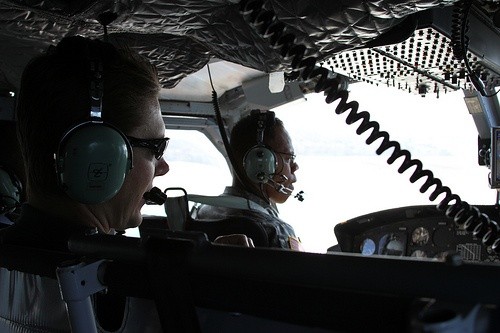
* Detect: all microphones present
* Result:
[143,187,167,205]
[258,174,306,202]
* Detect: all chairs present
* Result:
[139,187,290,249]
[0,229,202,333]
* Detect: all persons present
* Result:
[0,33,257,333]
[192,108,300,251]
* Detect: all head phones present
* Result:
[241,109,279,185]
[52,39,135,204]
